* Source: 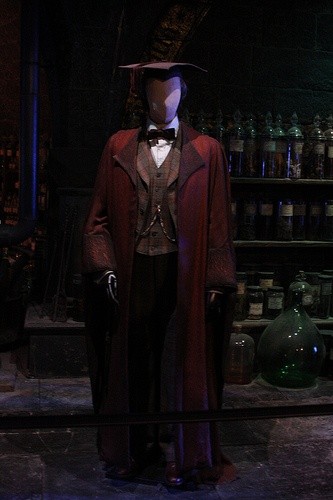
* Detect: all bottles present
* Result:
[256,289,327,389]
[182,107,333,179]
[220,324,256,385]
[232,192,333,240]
[236,270,333,320]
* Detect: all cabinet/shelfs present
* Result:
[0,132,333,379]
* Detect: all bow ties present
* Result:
[147,128,175,140]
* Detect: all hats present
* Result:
[119,60,209,113]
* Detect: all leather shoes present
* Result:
[114,462,129,475]
[166,460,185,486]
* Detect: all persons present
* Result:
[83,62,236,488]
